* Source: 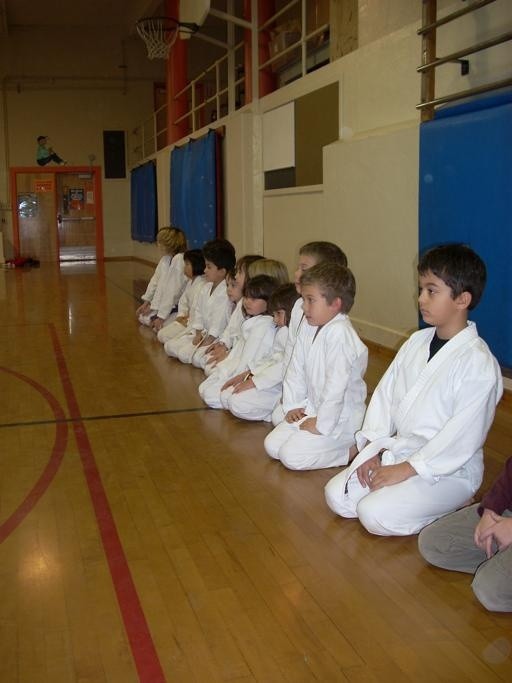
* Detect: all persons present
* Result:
[416,456,512,614]
[324,242,505,537]
[135,228,236,363]
[262,260,370,470]
[191,255,302,421]
[37,135,68,166]
